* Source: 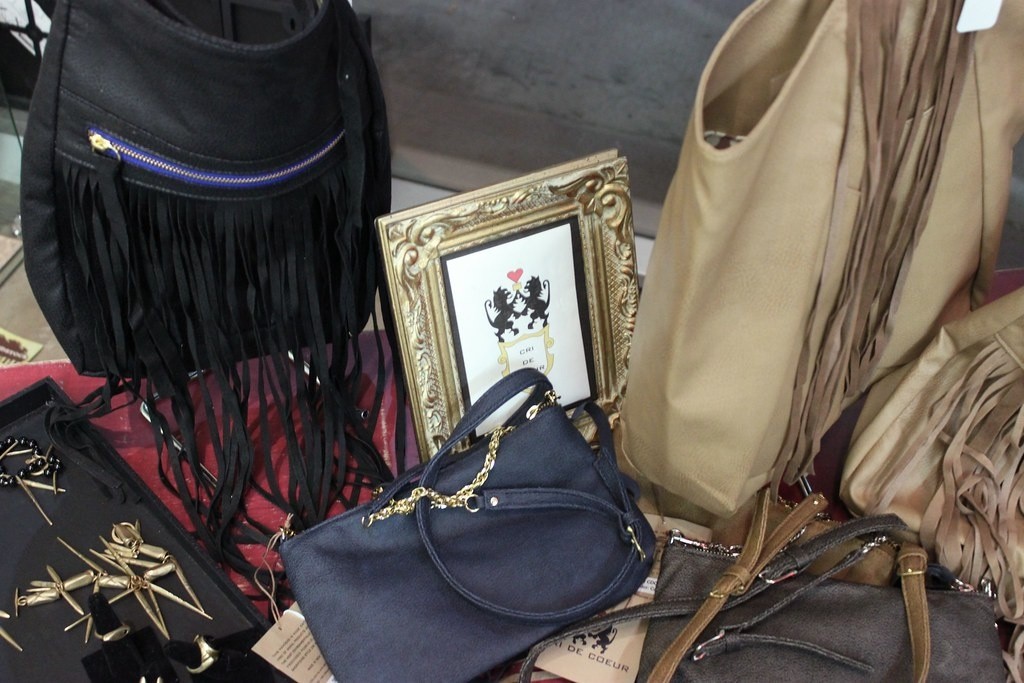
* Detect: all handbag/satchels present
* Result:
[517,513,1006,683]
[613,0,1024,518]
[279,363,654,683]
[840,287,1024,626]
[19,0,407,591]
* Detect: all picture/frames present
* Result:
[372,148,639,466]
